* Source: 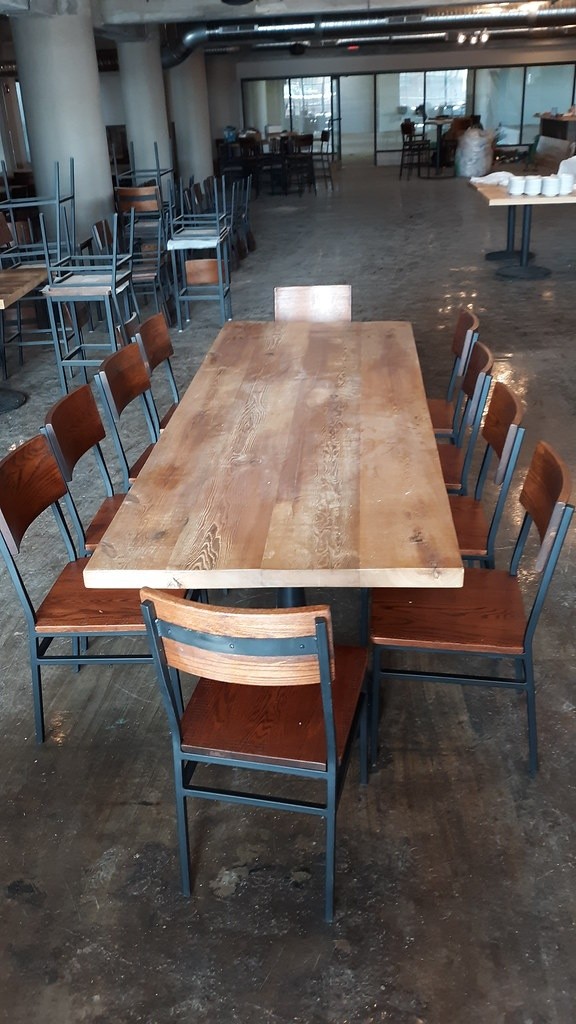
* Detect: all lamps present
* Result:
[446,26,490,45]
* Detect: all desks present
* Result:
[83,317,464,750]
[539,116,576,144]
[422,116,462,179]
[0,266,55,417]
[468,174,576,282]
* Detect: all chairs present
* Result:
[0,118,576,925]
